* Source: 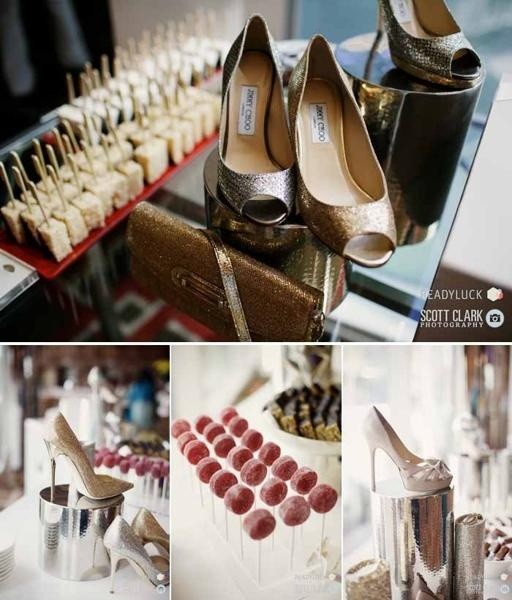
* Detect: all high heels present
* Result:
[375,1,483,91]
[131,505,170,557]
[91,512,112,569]
[103,512,170,597]
[364,405,454,496]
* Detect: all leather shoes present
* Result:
[216,12,296,227]
[43,411,135,503]
[289,32,397,270]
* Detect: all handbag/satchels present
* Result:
[125,202,325,341]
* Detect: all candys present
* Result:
[172,406,338,585]
[92,447,169,502]
